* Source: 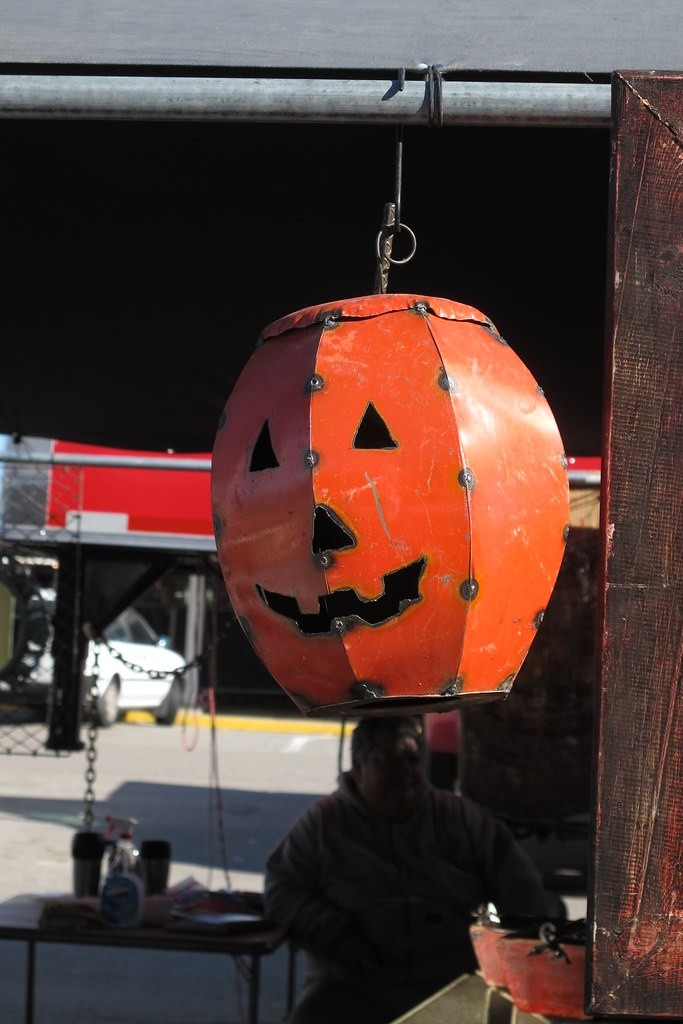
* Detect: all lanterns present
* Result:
[211,295,569,718]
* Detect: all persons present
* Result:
[265,718,565,1024]
[16,567,56,685]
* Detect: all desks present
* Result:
[0,893,299,1024]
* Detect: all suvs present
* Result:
[0,588,189,726]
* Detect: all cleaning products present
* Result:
[99,814,147,930]
